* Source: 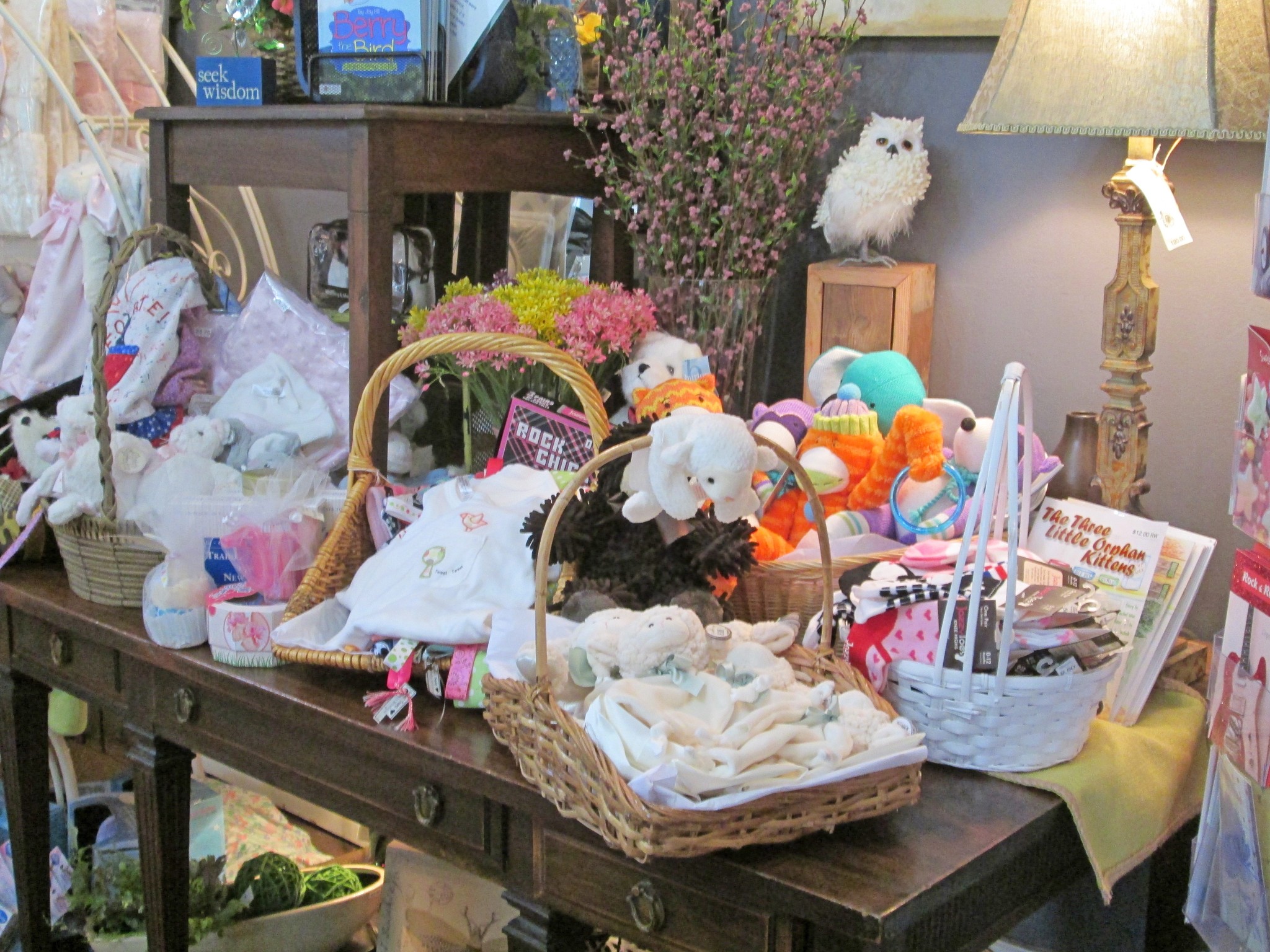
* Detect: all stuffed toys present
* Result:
[752,399,817,495]
[124,414,235,518]
[621,406,778,524]
[811,109,931,270]
[767,345,928,493]
[516,604,707,701]
[214,417,301,472]
[10,329,1062,626]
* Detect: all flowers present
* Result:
[397,265,656,432]
[508,0,878,409]
[180,0,294,51]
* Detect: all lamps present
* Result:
[955,0,1270,519]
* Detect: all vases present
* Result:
[259,36,308,101]
[1046,411,1109,505]
[644,272,780,422]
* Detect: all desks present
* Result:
[1,558,1213,952]
[134,100,634,485]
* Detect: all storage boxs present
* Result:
[194,56,278,107]
[802,258,938,406]
[496,389,618,474]
[0,785,68,858]
[844,598,997,672]
[114,765,226,873]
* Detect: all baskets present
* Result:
[0,477,45,554]
[481,431,922,862]
[728,547,902,631]
[882,363,1123,774]
[53,225,220,606]
[270,331,610,672]
[40,722,228,876]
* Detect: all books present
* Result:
[316,0,508,103]
[1027,497,1216,725]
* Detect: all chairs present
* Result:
[45,686,88,809]
